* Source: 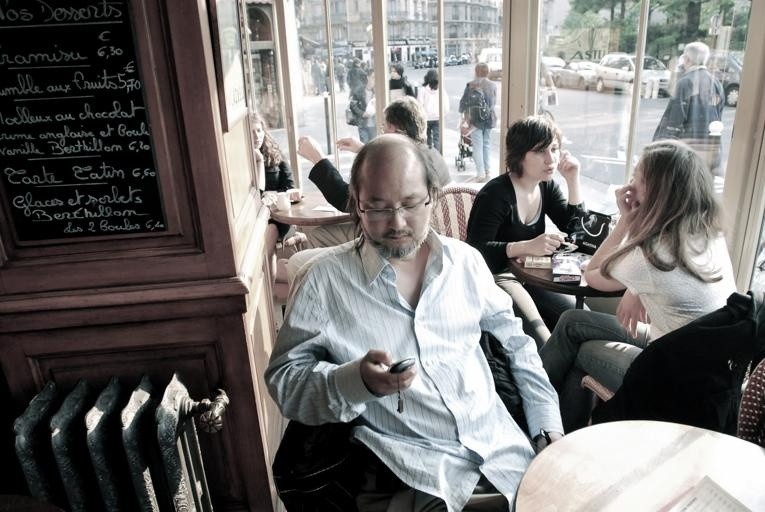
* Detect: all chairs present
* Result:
[576,293,755,433]
[423,189,489,249]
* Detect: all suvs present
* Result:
[675,51,743,107]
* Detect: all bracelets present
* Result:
[507,241,515,257]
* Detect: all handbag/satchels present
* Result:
[576,210,611,257]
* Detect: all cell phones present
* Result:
[389,358,415,372]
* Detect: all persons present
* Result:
[299,55,366,97]
[652,39,726,189]
[264,133,566,512]
[459,61,498,182]
[416,53,472,70]
[539,60,557,114]
[346,63,449,151]
[540,137,739,434]
[464,114,591,348]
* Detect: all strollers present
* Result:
[455,110,476,171]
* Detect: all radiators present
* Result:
[1,372,232,510]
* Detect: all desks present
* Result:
[514,245,626,317]
[262,186,375,227]
[516,417,765,509]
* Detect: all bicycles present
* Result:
[538,85,556,123]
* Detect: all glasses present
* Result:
[356,191,432,223]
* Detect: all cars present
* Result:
[541,56,565,79]
[413,53,471,70]
[553,59,599,90]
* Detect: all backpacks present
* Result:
[466,79,494,122]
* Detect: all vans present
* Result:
[477,47,502,81]
[596,52,675,98]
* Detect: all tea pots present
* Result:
[270,192,291,211]
